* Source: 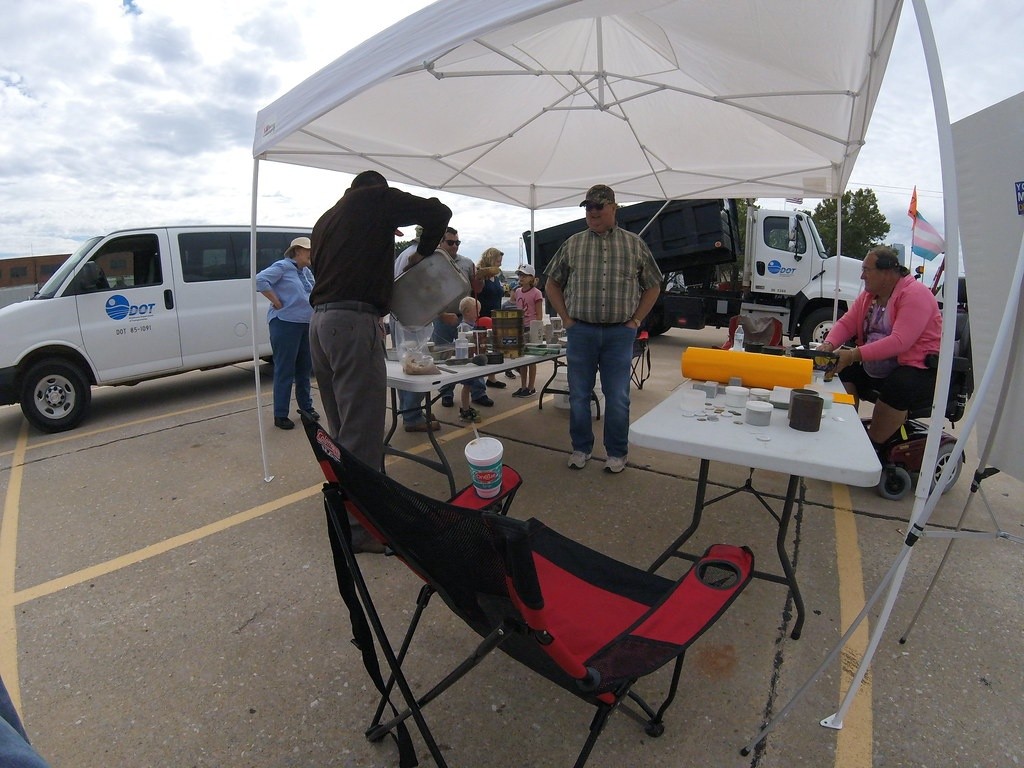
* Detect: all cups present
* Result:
[788,395,824,432]
[787,389,819,419]
[550,317,562,330]
[465,436,503,499]
[427,342,434,352]
[454,339,468,359]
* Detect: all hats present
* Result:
[580,185,616,207]
[515,263,535,277]
[284,236,311,258]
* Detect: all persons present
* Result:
[388,226,542,431]
[255,237,320,429]
[815,245,943,451]
[542,185,663,473]
[308,171,452,554]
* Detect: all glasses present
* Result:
[861,267,878,274]
[586,202,614,211]
[519,273,532,277]
[443,240,461,246]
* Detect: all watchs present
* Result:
[632,317,641,328]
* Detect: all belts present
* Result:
[573,318,627,328]
[312,299,380,318]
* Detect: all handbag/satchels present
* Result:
[395,320,441,375]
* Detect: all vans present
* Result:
[1,225,317,437]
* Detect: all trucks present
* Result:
[523,197,868,358]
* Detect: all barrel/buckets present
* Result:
[553,366,571,409]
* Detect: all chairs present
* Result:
[295,409,755,767]
[144,256,156,284]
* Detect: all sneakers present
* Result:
[471,395,494,408]
[442,395,454,407]
[273,416,296,429]
[603,454,629,474]
[459,408,482,422]
[304,408,320,418]
[567,449,592,469]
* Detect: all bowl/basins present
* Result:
[725,385,749,408]
[745,342,764,353]
[681,389,707,412]
[762,345,784,356]
[744,401,774,426]
[791,349,839,370]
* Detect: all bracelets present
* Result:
[850,346,859,367]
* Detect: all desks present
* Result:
[381,336,600,498]
[628,347,882,640]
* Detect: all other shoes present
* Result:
[423,412,436,421]
[403,420,441,432]
[513,367,521,374]
[505,370,516,378]
[519,387,536,397]
[512,388,528,397]
[486,379,507,388]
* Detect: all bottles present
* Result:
[733,325,744,352]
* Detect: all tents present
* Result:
[250,1,1024,729]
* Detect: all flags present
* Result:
[908,186,916,231]
[912,210,945,261]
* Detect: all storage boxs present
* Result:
[530,319,542,343]
[490,309,526,358]
